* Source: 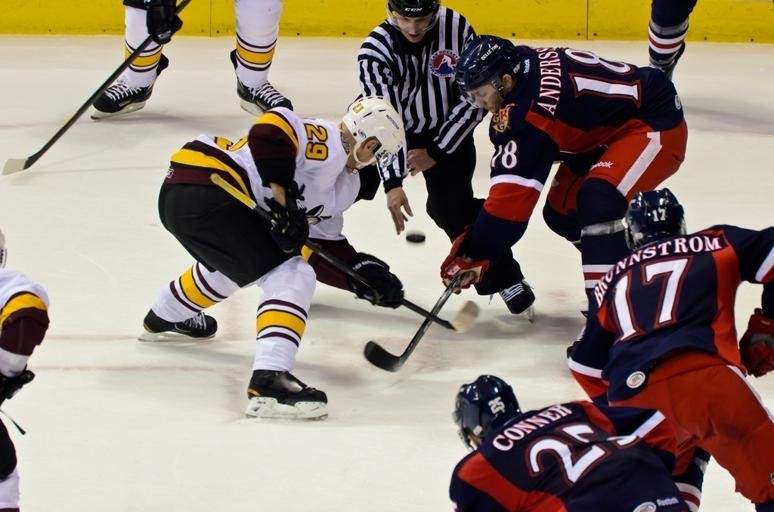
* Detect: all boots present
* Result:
[89,52,168,118]
[229,50,293,112]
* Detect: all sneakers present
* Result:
[142,311,217,339]
[498,276,534,315]
[246,369,326,411]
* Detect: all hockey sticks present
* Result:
[366,274,468,372]
[4,2,191,176]
[208,175,480,332]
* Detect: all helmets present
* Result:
[452,376,521,444]
[341,95,405,169]
[385,0,440,20]
[453,35,520,93]
[620,187,687,250]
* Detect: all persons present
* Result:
[648,0,696,81]
[357,1,535,312]
[568,190,773,512]
[90,0,293,111]
[0,230,48,512]
[144,95,410,406]
[451,374,690,512]
[441,34,687,357]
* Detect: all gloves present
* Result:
[263,180,309,257]
[145,0,183,44]
[346,251,404,309]
[739,305,774,377]
[440,225,491,295]
